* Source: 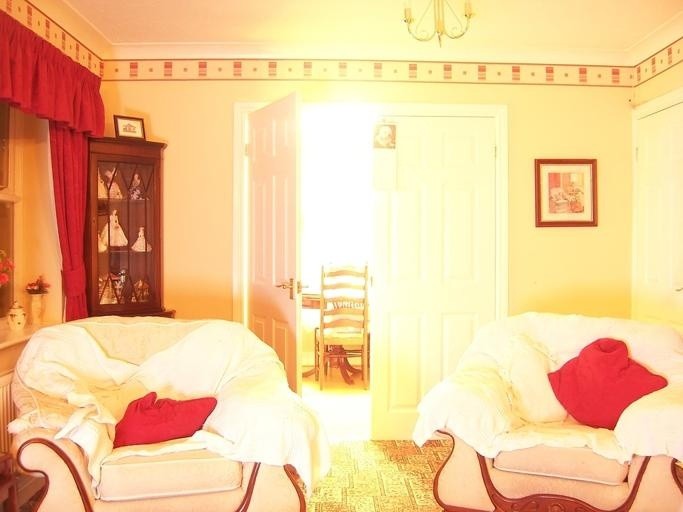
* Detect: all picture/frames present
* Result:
[533,156,600,230]
[112,113,149,140]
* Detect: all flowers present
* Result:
[1,249,15,318]
[26,276,50,295]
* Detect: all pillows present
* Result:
[498,329,667,433]
[107,391,217,449]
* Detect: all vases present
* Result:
[30,294,42,325]
[8,306,26,330]
[0,317,10,342]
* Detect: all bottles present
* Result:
[9,301,26,330]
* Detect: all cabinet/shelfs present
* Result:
[84,134,173,318]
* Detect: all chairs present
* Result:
[314,263,371,393]
[421,308,682,512]
[11,312,314,512]
